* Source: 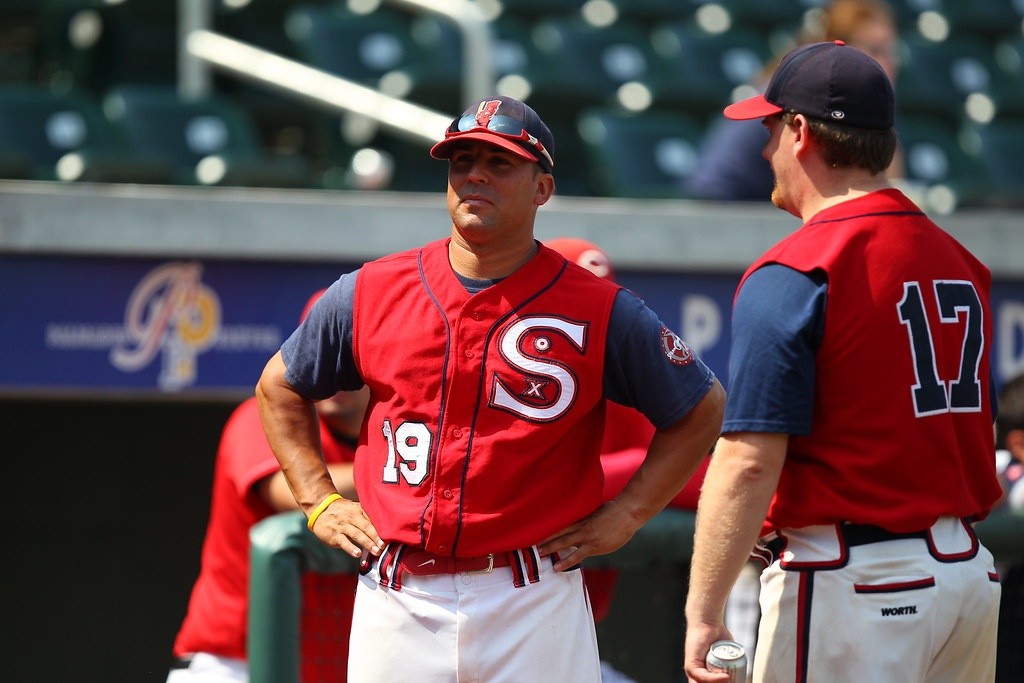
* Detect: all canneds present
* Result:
[705,638,748,683]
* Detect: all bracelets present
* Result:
[307,493,341,531]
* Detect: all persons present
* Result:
[519,234,661,634]
[254,93,729,683]
[160,286,374,683]
[680,37,1005,683]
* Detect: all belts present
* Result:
[400,547,511,577]
[764,521,927,570]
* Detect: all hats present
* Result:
[430,95,555,174]
[723,39,896,130]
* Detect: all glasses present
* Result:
[445,113,554,168]
